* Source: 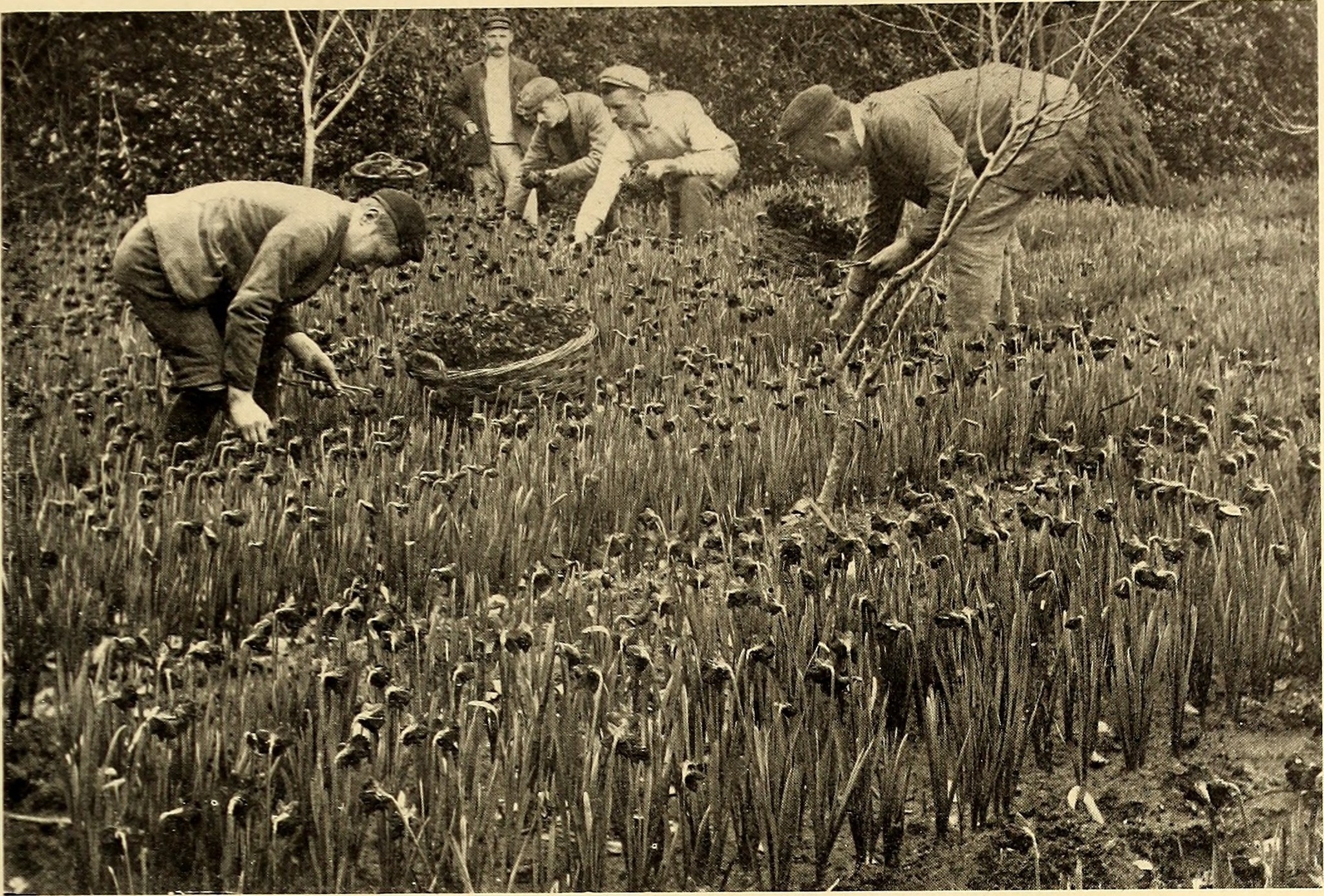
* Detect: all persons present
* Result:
[771,63,1089,345]
[568,63,740,248]
[438,15,541,219]
[112,181,425,467]
[503,77,621,228]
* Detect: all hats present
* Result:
[778,85,842,159]
[372,188,426,264]
[484,15,511,30]
[515,76,557,114]
[598,64,652,93]
